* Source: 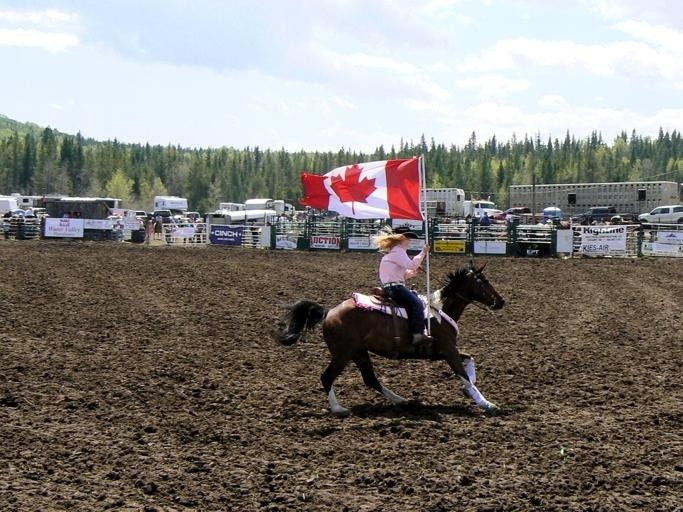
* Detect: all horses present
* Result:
[270,259,504,417]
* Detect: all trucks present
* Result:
[422,188,503,219]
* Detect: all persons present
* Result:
[373,226,433,346]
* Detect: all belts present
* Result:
[384,282,405,287]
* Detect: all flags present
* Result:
[299,156,428,221]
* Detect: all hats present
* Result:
[387,226,418,239]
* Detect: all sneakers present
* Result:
[412,333,434,345]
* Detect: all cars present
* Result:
[498,207,530,215]
[135,210,203,223]
[7,207,45,218]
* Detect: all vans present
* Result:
[639,205,683,225]
[577,207,616,222]
